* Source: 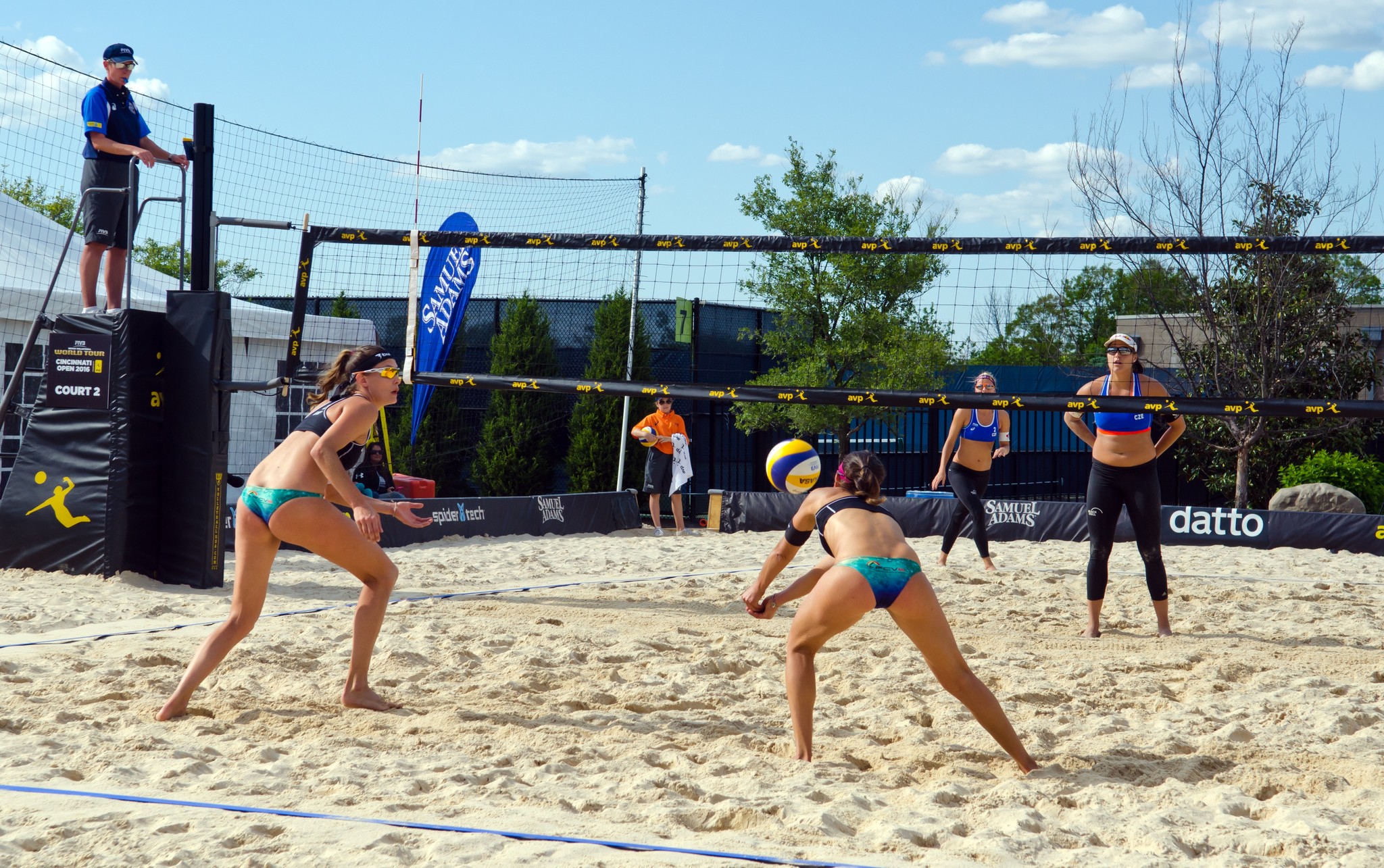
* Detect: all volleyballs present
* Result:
[766,438,821,494]
[638,426,659,447]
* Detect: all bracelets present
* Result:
[393,502,397,518]
[771,594,781,608]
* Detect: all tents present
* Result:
[0,197,378,506]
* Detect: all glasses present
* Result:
[1107,347,1136,355]
[659,400,672,405]
[975,384,994,391]
[371,450,383,455]
[352,367,400,378]
[103,57,136,70]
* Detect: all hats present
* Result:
[1104,333,1137,352]
[655,397,672,401]
[103,43,138,65]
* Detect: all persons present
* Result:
[630,397,701,537]
[1063,333,1186,638]
[929,372,1009,578]
[81,42,190,318]
[154,348,434,712]
[742,454,1041,774]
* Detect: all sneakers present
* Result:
[676,527,701,536]
[654,527,663,537]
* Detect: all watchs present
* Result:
[168,153,179,161]
